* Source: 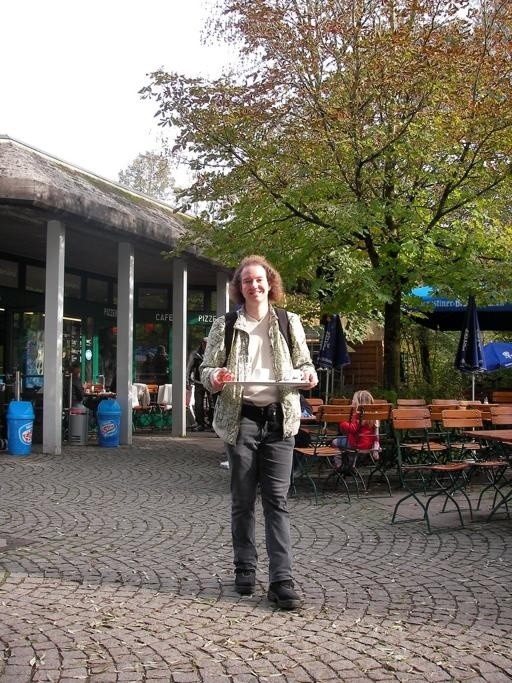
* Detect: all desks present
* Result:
[83,390,118,442]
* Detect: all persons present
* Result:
[185,337,215,433]
[67,368,88,409]
[331,390,380,485]
[138,344,169,387]
[292,391,313,474]
[198,254,319,610]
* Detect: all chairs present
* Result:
[131,382,201,434]
[289,390,512,536]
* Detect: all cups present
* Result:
[290,369,303,380]
[238,355,252,381]
[256,368,269,380]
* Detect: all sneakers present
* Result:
[267,580,300,609]
[234,568,255,594]
[193,424,206,432]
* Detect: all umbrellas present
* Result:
[311,266,351,429]
[482,342,512,375]
[454,295,487,456]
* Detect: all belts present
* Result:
[241,406,281,422]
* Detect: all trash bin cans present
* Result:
[96,400,122,447]
[6,401,35,455]
